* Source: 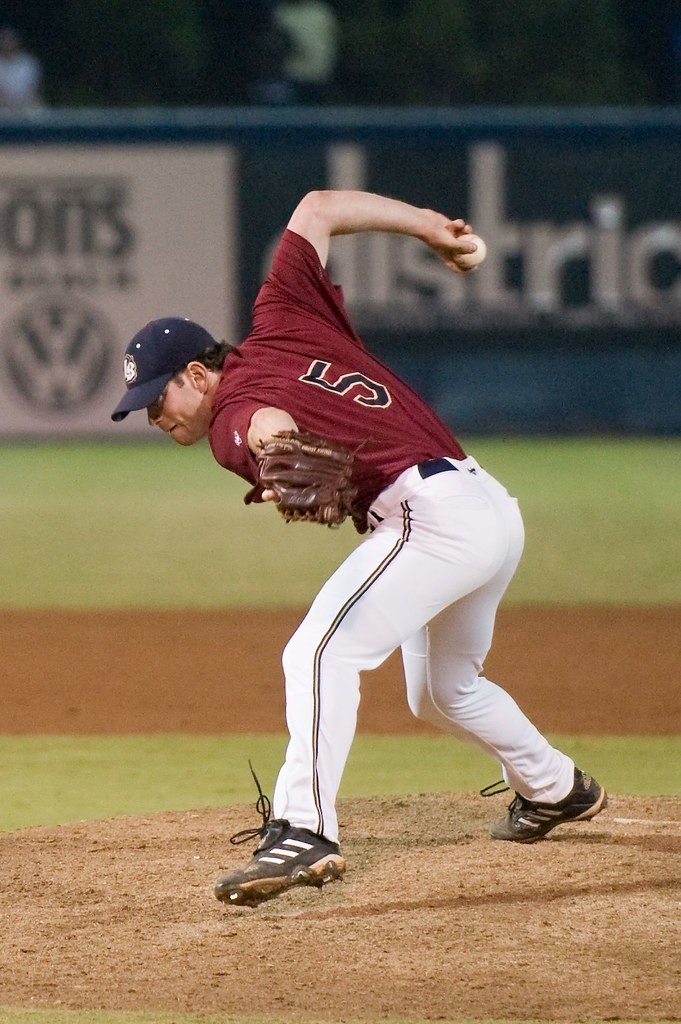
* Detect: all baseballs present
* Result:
[454,233,487,269]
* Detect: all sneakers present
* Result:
[480,767,609,843]
[214,760,346,908]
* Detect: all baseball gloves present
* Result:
[254,425,361,528]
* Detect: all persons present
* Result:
[0,0,335,112]
[112,191,606,906]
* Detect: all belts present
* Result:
[368,458,460,522]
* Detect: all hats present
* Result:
[110,317,216,422]
